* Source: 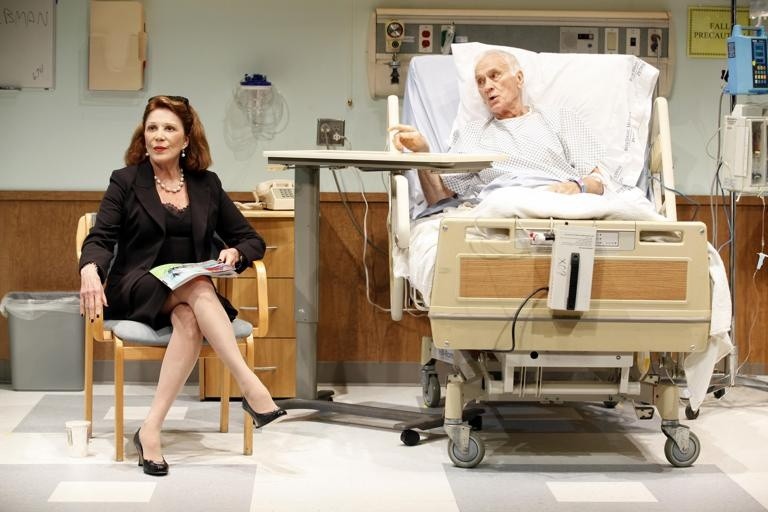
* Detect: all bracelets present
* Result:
[568,177,587,193]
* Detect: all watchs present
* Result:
[237,250,243,271]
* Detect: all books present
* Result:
[148,259,238,292]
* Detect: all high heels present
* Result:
[240,387,289,430]
[131,422,172,476]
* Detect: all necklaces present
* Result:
[153,166,185,194]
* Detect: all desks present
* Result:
[263,147,504,443]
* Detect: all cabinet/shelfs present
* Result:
[199,203,299,405]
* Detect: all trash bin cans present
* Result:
[6,291,85,391]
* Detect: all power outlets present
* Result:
[315,118,346,149]
[647,28,663,58]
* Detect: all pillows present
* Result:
[445,41,662,188]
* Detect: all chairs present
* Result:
[76,210,271,460]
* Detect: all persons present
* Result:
[385,50,606,210]
[78,95,288,476]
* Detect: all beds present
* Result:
[386,41,729,472]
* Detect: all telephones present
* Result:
[257,178,295,210]
